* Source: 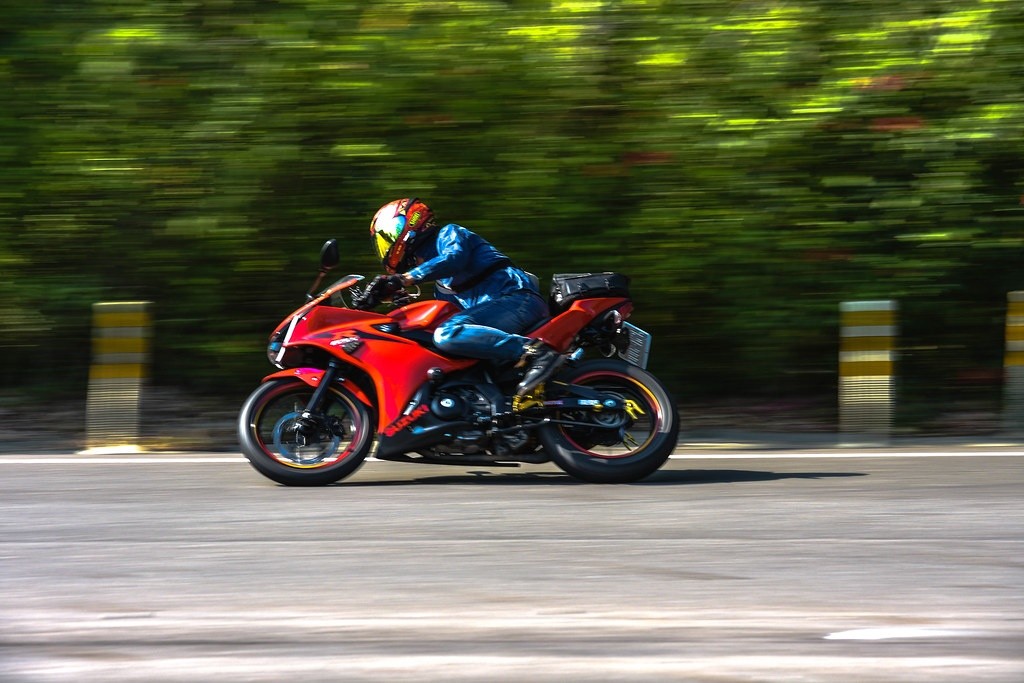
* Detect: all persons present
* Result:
[366,198,566,395]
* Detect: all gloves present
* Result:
[365,275,402,303]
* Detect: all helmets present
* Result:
[370,197,434,273]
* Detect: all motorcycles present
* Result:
[237,238,680,488]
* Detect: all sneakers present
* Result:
[518,340,568,392]
